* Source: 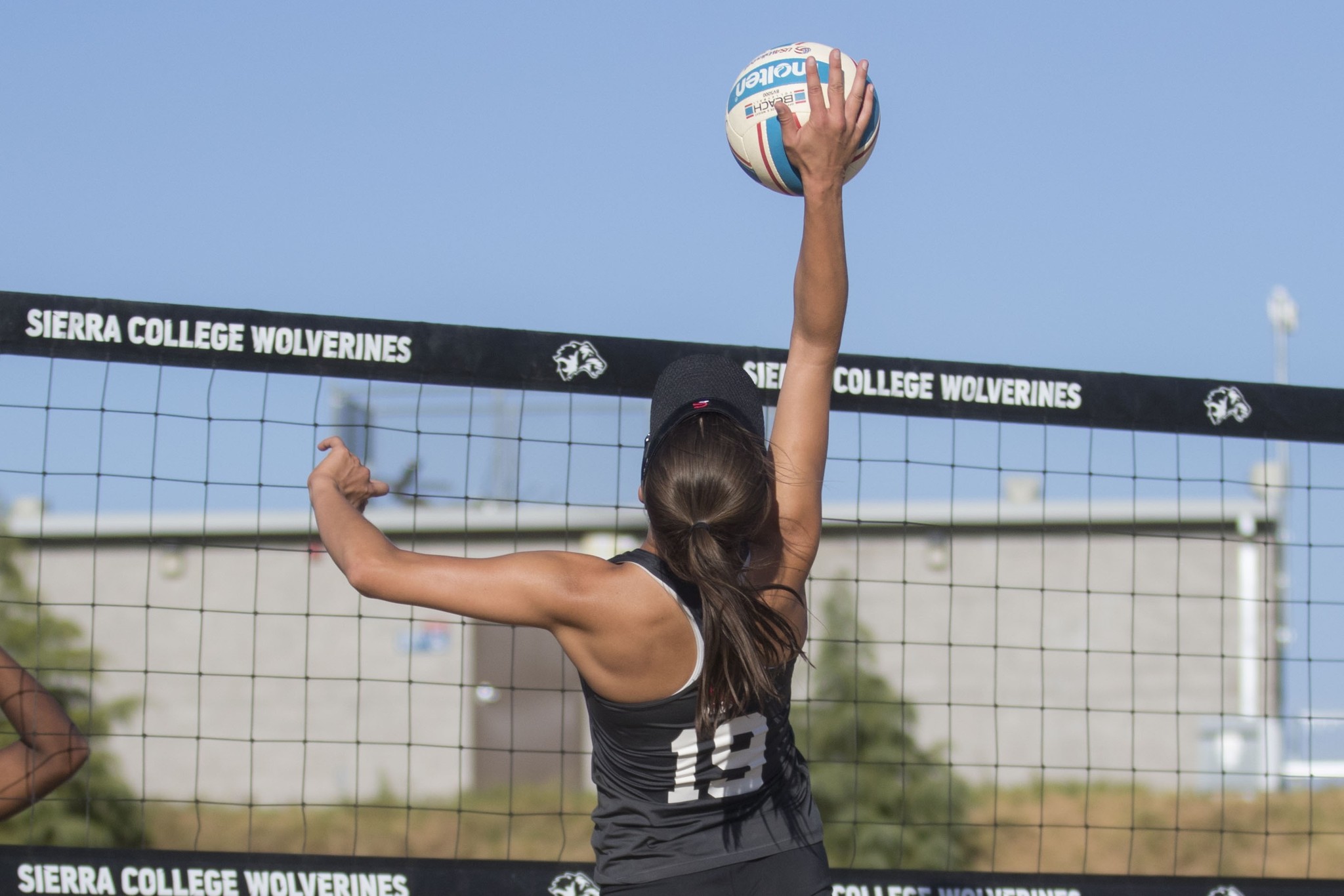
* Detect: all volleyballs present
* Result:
[725,40,881,198]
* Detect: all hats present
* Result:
[640,353,764,494]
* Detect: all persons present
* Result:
[0,648,92,822]
[309,46,871,896]
